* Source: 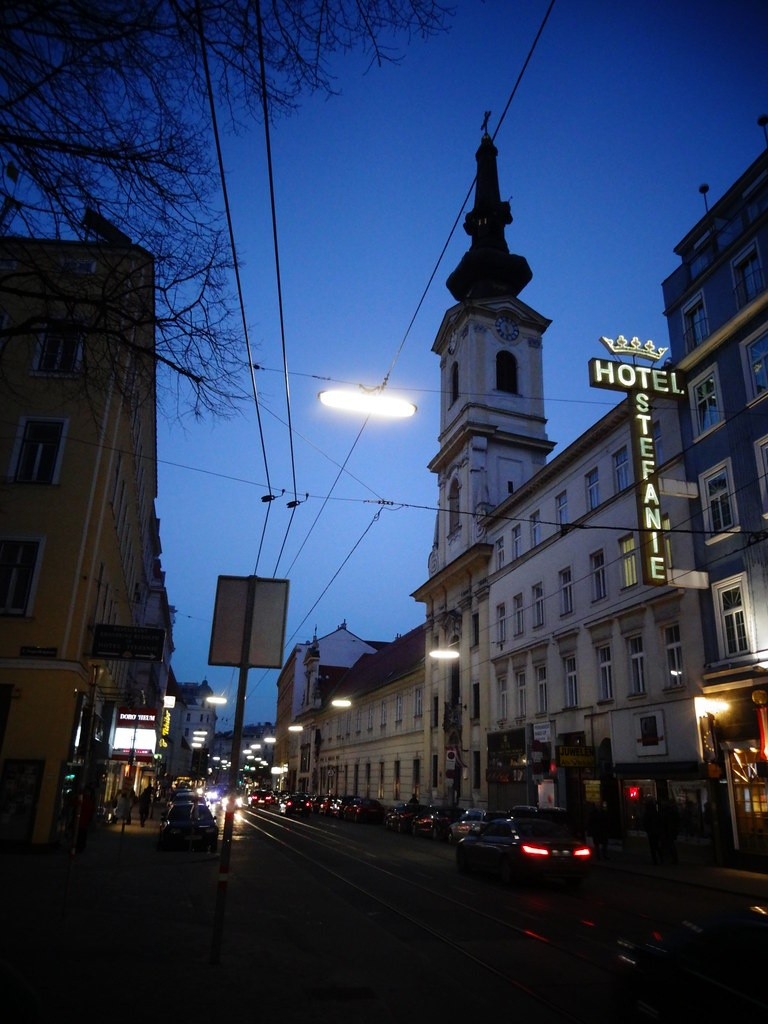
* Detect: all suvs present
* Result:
[510,805,567,816]
[448,806,508,846]
[411,807,465,838]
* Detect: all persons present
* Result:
[138,783,154,827]
[408,793,419,804]
[111,787,135,825]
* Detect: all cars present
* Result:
[272,790,384,825]
[385,803,426,832]
[158,783,218,855]
[456,817,591,882]
[247,790,273,808]
